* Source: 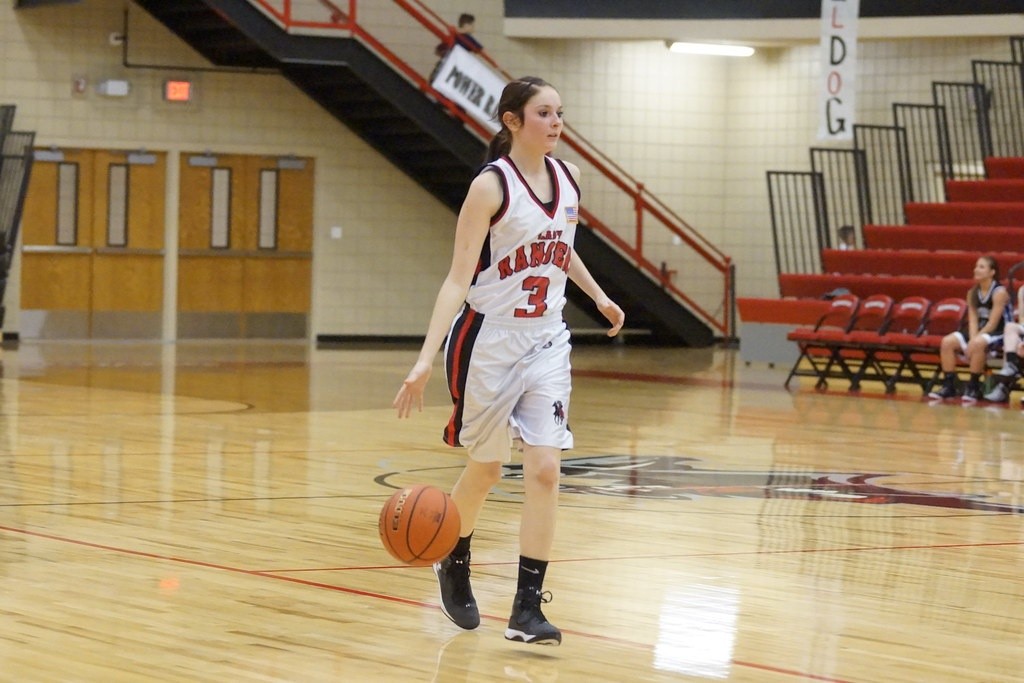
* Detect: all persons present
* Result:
[837,226,854,251]
[928,257,1015,401]
[983,284,1024,402]
[935,405,1024,518]
[393,75,625,645]
[435,13,503,127]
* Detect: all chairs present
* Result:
[784,292,968,395]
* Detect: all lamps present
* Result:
[98,77,134,98]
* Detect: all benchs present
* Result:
[729,155,1024,328]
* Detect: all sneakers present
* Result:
[431,632,480,683]
[432,550,480,630]
[506,585,561,646]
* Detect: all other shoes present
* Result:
[961,380,984,402]
[927,385,960,400]
[983,382,1009,402]
